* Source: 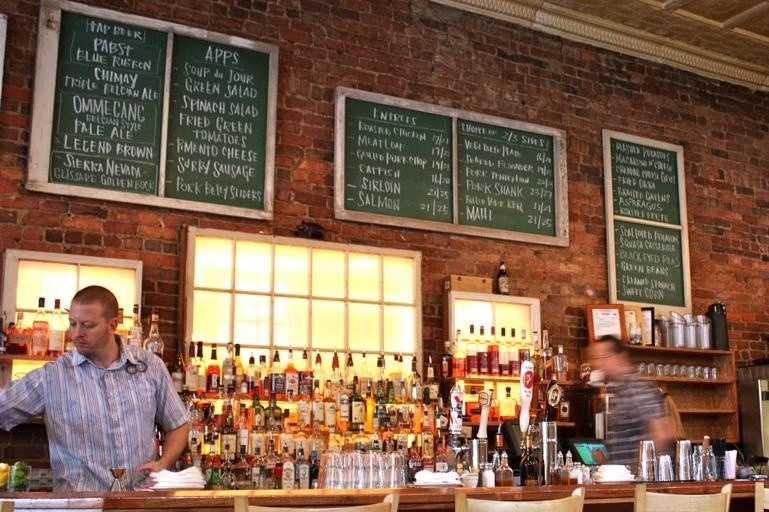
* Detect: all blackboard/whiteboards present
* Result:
[24,0,280,221]
[333,87,570,248]
[602,128,693,321]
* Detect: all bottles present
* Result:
[407,431,450,487]
[455,324,569,382]
[116,302,163,361]
[155,341,452,458]
[709,303,730,351]
[450,382,593,486]
[181,435,319,489]
[496,259,511,295]
[2,296,77,360]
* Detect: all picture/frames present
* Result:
[585,302,656,348]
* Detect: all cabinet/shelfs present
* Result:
[591,344,739,451]
[0,348,64,429]
[158,389,450,478]
[444,371,576,463]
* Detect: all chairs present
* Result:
[234,492,400,512]
[633,483,733,512]
[755,482,769,512]
[757,379,769,458]
[455,486,586,512]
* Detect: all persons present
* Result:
[585,335,679,466]
[0,286,190,494]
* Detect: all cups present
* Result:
[629,320,643,344]
[635,361,720,379]
[319,448,407,489]
[109,466,125,491]
[1,464,32,492]
[635,432,737,482]
[656,311,711,350]
